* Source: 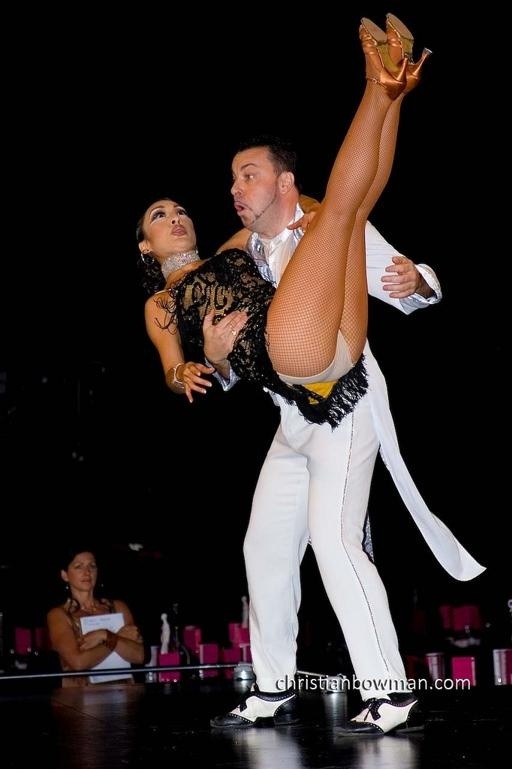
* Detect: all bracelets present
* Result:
[101,629,120,651]
[170,362,185,388]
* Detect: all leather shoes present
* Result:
[209,683,309,731]
[332,695,429,738]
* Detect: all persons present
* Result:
[134,11,434,402]
[201,141,428,738]
[159,613,171,655]
[238,596,249,630]
[45,546,144,685]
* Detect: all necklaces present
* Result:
[154,249,202,279]
[80,601,100,614]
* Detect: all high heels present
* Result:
[385,11,433,97]
[357,16,412,101]
